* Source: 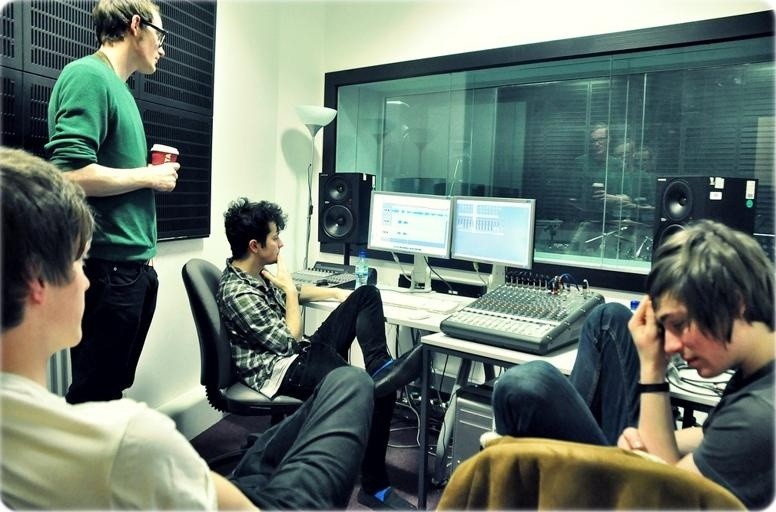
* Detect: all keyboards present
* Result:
[378,290,460,314]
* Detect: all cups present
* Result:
[149,142,180,165]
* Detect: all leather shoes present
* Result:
[371,346,424,395]
[355,487,416,512]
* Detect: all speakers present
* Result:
[318,172,376,245]
[652,176,759,264]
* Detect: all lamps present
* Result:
[294,105,338,269]
[363,117,395,187]
[407,126,437,193]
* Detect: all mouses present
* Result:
[406,309,432,320]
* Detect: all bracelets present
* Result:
[636,382,670,393]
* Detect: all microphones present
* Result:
[453,153,471,161]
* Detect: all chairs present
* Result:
[182,259,303,476]
[434,434,751,511]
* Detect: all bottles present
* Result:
[354,250,369,292]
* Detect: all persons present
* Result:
[0,146,375,512]
[492,219,776,512]
[44,0,180,405]
[572,123,656,256]
[216,197,430,512]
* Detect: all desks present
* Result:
[300,282,736,511]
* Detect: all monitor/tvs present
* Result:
[367,191,454,293]
[450,196,537,293]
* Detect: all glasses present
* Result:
[141,18,167,47]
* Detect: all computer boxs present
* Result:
[451,376,500,476]
